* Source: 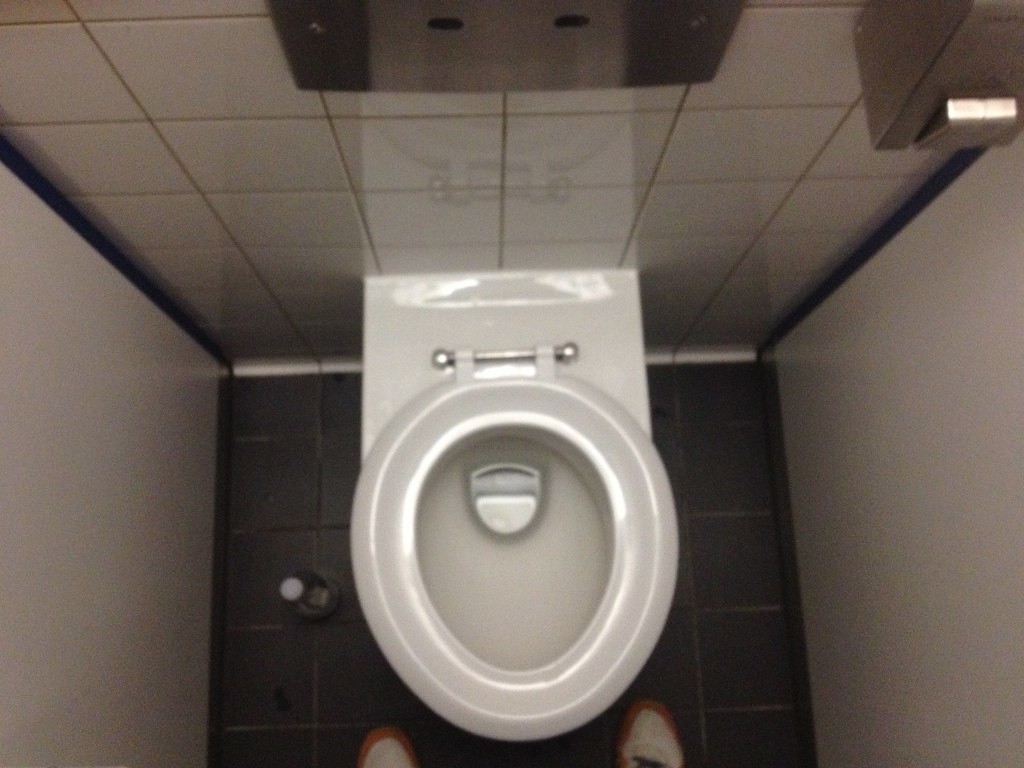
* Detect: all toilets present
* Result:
[348,269,681,748]
[360,4,640,269]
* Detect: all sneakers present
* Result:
[355,724,419,767]
[611,697,683,768]
